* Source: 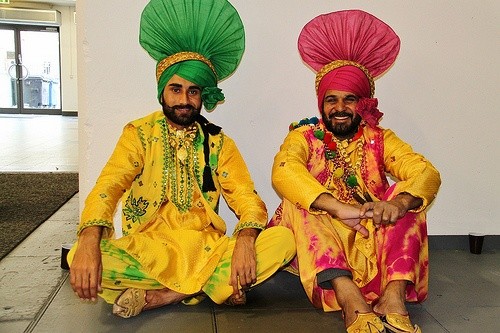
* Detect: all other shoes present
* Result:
[225,290,248,307]
[376,312,422,333]
[113,288,149,318]
[342,309,385,333]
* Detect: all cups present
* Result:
[468,234,485,255]
[60,242,74,270]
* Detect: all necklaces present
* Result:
[166,124,200,209]
[312,122,362,204]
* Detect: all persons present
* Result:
[67,0,295,319]
[268,10,441,333]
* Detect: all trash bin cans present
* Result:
[42,78,53,106]
[11,76,42,107]
